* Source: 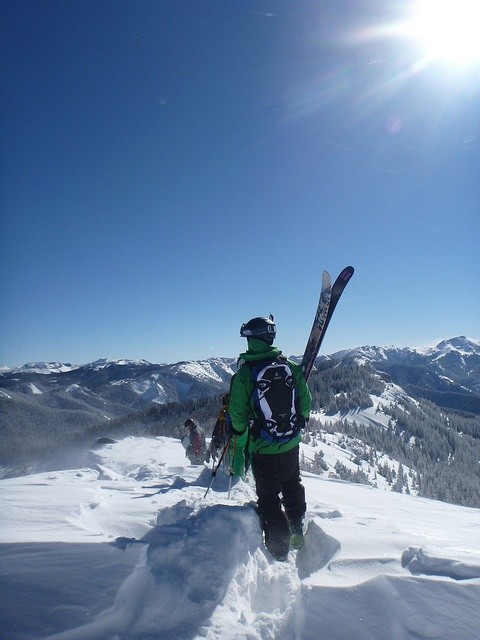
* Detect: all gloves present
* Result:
[229,420,247,438]
[212,449,219,462]
[205,450,210,463]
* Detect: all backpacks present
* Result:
[212,409,227,448]
[244,355,306,455]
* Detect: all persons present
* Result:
[212,393,251,475]
[228,316,313,533]
[181,418,205,466]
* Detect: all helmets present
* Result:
[240,312,276,345]
[184,418,197,430]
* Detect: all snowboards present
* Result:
[181,435,191,451]
